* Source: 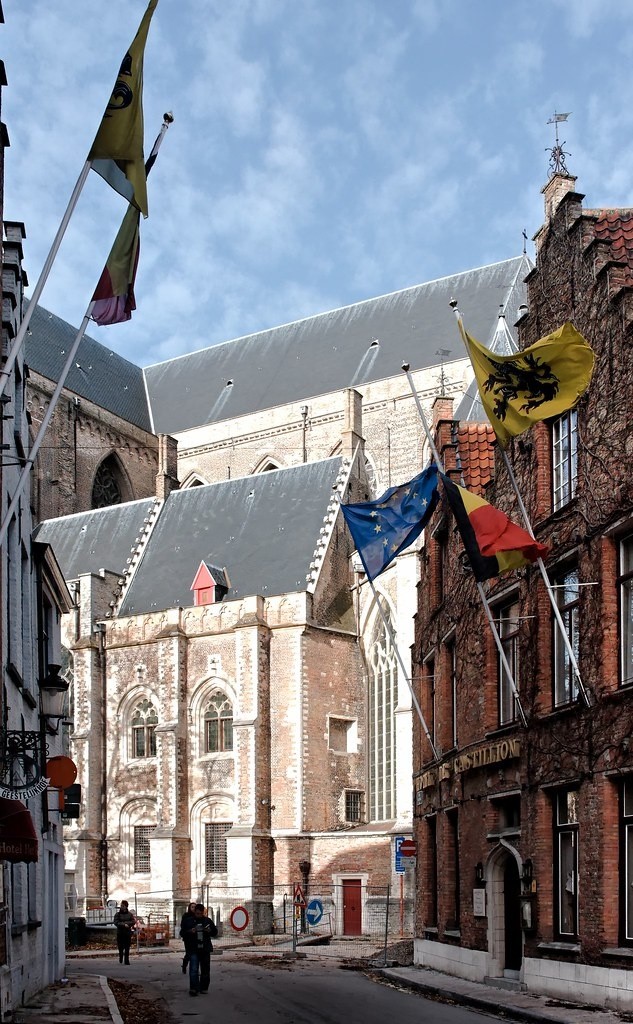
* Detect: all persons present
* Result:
[114,900,135,966]
[180,903,218,996]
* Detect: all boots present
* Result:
[182,959,188,974]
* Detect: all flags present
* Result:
[341,464,439,582]
[89,153,157,326]
[86,0,149,219]
[440,471,549,584]
[465,322,595,441]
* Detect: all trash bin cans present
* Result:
[68,916,86,946]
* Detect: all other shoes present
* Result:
[200,990,208,994]
[189,990,198,996]
[124,960,130,965]
[119,955,123,963]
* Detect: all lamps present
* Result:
[475,862,485,887]
[523,858,533,884]
[41,664,69,733]
